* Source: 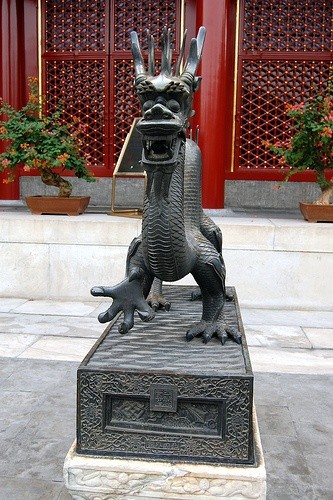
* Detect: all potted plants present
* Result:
[261,81,333,223]
[0,76,96,216]
